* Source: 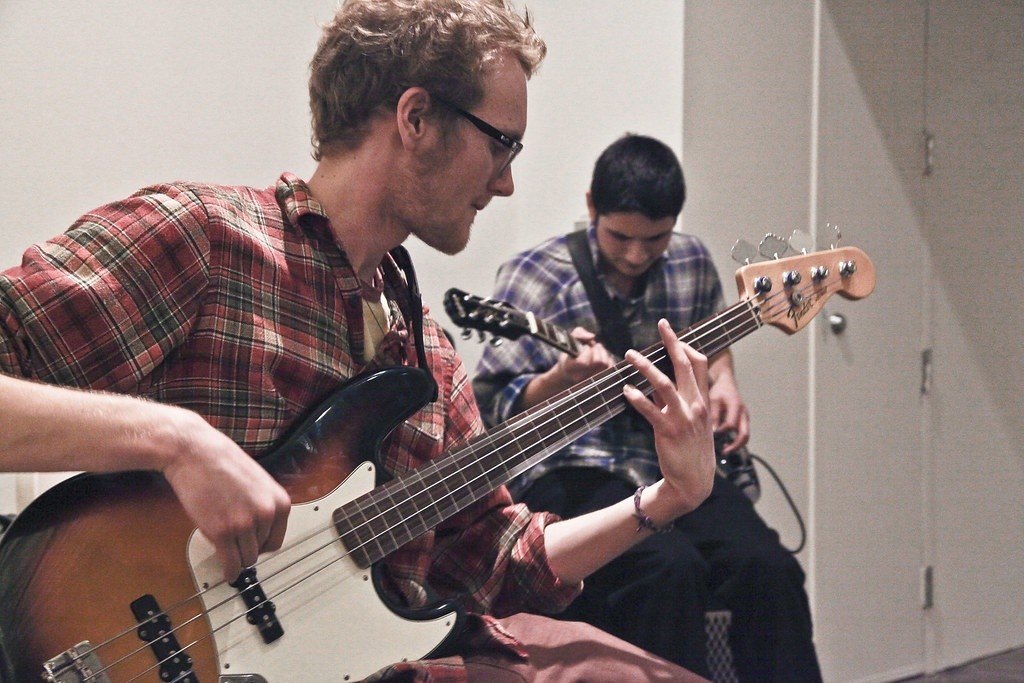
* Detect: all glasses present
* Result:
[437,89,523,178]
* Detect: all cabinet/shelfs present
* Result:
[682,1,1024,683]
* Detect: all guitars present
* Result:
[1,223,879,682]
[442,283,762,506]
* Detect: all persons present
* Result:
[472,134,822,683]
[0,0,716,683]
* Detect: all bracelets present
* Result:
[627,482,668,537]
